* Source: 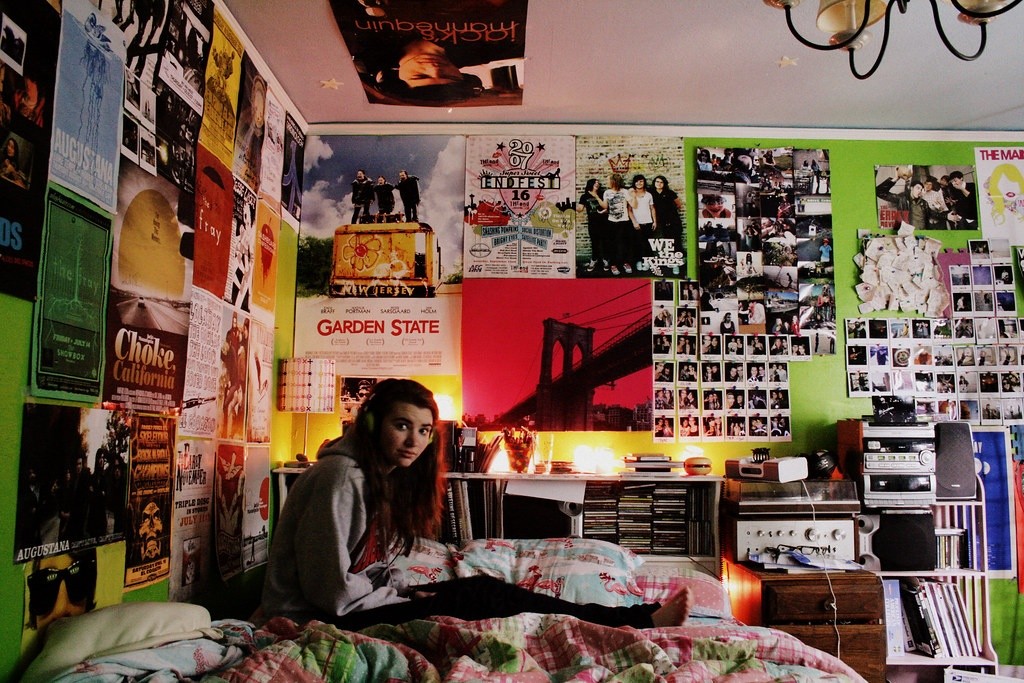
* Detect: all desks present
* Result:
[272,468,726,580]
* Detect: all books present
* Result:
[442,453,713,557]
[885,503,984,658]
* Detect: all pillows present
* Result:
[389,533,646,608]
[632,567,732,619]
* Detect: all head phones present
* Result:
[360,389,435,444]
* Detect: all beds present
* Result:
[15,596,868,683]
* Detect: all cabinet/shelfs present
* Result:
[722,553,886,683]
[869,473,998,683]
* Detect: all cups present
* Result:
[531,432,555,474]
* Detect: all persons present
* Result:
[343,149,1024,438]
[383,40,480,104]
[266,378,693,633]
[0,0,266,635]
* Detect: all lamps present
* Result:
[762,0,1023,80]
[278,358,337,468]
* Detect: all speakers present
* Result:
[934,422,977,500]
